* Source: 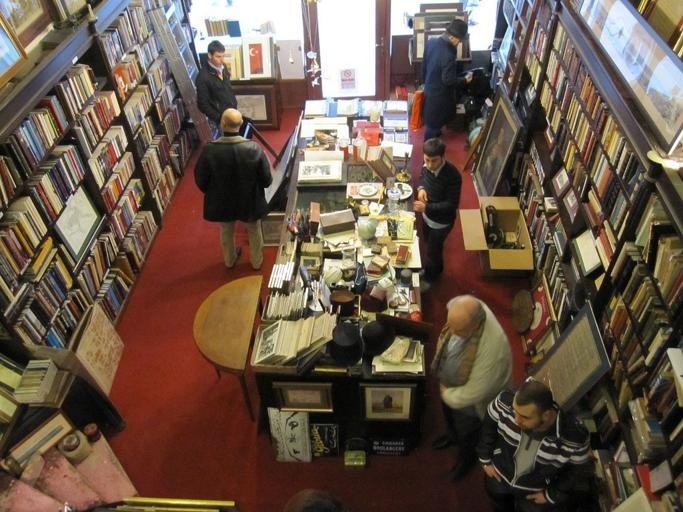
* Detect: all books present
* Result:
[513,113,683,510]
[202,15,244,38]
[481,1,681,114]
[1,0,201,409]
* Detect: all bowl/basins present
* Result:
[329,289,355,315]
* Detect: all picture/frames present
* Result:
[231,84,279,131]
[122,496,235,508]
[470,84,524,208]
[115,506,220,512]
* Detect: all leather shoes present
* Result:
[224,246,244,270]
[449,458,472,485]
[430,432,460,451]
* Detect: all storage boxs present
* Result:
[459,197,535,283]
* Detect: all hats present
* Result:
[444,18,469,41]
[362,320,397,358]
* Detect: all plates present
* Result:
[358,183,412,201]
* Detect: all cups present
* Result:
[337,141,361,162]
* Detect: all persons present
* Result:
[411,137,462,296]
[381,390,393,411]
[420,20,474,141]
[475,380,595,511]
[196,40,238,138]
[430,293,512,481]
[479,124,507,180]
[192,107,273,273]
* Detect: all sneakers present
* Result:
[418,275,439,295]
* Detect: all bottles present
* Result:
[83,422,100,442]
[400,269,412,284]
[63,434,79,451]
[388,187,400,212]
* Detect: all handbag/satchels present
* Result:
[410,87,427,134]
